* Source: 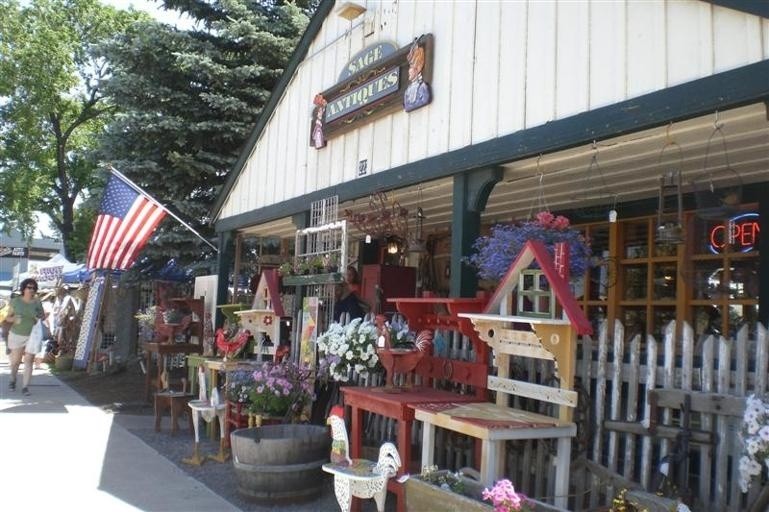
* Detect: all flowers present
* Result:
[460,209,593,290]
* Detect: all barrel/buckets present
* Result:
[230,424,333,505]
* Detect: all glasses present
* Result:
[24,285,36,290]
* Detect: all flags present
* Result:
[85,170,165,274]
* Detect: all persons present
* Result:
[53,284,77,354]
[5,278,45,396]
[345,266,361,298]
[402,47,432,113]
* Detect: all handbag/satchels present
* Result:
[24,316,45,356]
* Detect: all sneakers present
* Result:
[22,387,31,396]
[7,379,17,392]
